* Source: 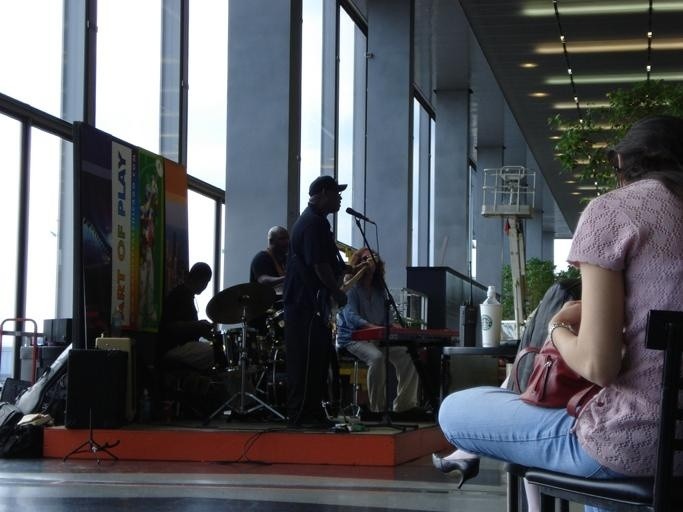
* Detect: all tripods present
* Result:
[63,409,118,463]
[203,328,287,424]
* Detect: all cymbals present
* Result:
[207,283,276,322]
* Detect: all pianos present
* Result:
[355,327,452,344]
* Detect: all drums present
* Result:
[215,326,270,375]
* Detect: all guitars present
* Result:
[313,257,377,327]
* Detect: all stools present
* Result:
[336,343,365,419]
[166,365,202,420]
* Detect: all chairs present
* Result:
[499,279,682,512]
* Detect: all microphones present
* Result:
[346,205,374,225]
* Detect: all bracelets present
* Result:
[548,320,577,348]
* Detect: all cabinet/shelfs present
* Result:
[405,265,519,394]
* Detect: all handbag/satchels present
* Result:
[512,336,602,416]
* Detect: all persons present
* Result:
[427,278,582,512]
[281,173,369,427]
[138,173,158,325]
[333,245,433,419]
[244,224,291,332]
[156,261,240,428]
[438,111,682,511]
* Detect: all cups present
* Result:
[478,303,503,348]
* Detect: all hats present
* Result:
[607,115,683,162]
[309,176,347,196]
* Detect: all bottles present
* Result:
[481,285,500,305]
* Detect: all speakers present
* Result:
[65,349,127,428]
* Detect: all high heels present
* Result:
[432,452,480,489]
